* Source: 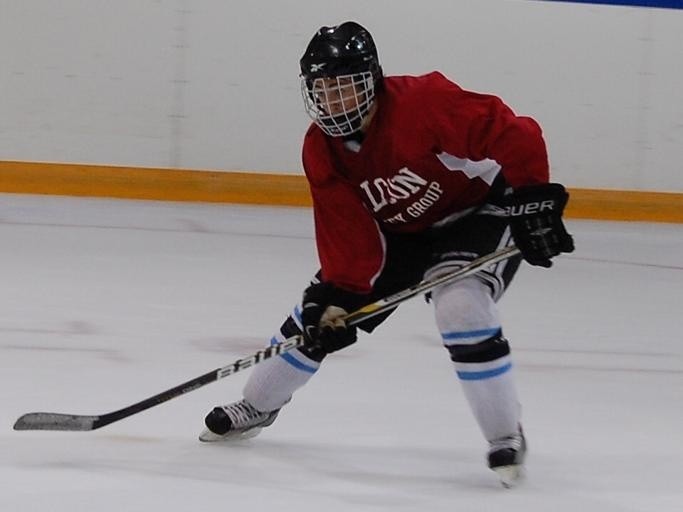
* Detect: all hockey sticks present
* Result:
[13,245,522,432]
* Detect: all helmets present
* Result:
[298,21,383,139]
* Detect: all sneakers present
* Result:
[487,421,526,467]
[204,397,280,435]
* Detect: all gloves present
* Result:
[299,281,356,354]
[501,184,575,269]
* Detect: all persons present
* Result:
[204,20,577,471]
